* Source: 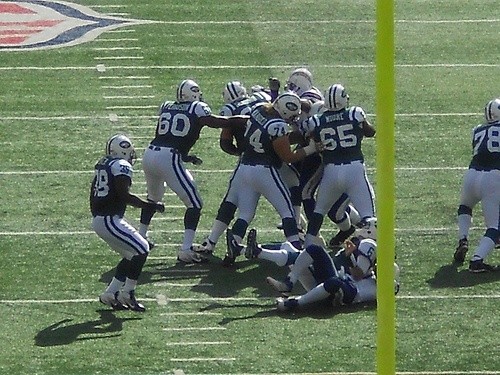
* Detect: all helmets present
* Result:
[249,92,272,109]
[309,101,327,115]
[355,217,377,240]
[288,67,313,92]
[324,84,347,110]
[300,99,313,117]
[222,81,247,104]
[273,92,301,120]
[176,80,202,103]
[485,98,500,122]
[105,133,136,165]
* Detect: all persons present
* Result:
[138,79,251,262]
[190,68,400,309]
[454,99,500,272]
[90,135,165,312]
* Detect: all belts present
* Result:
[476,168,490,171]
[335,160,363,165]
[149,146,175,153]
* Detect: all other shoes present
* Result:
[330,225,356,245]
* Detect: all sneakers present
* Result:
[117,291,145,311]
[454,240,468,261]
[468,259,491,272]
[99,291,128,311]
[276,295,297,311]
[175,230,263,266]
[267,277,292,297]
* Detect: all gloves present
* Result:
[152,202,165,212]
[191,155,202,165]
